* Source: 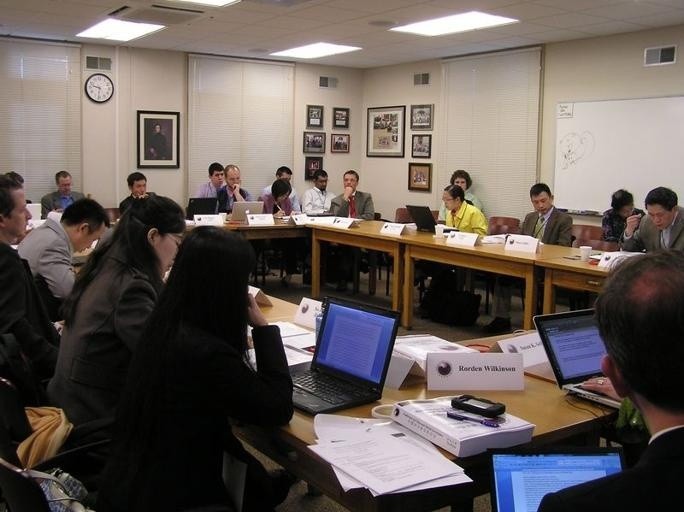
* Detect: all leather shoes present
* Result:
[336,280,346,291]
[481,317,511,334]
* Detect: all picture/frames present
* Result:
[85,72,114,104]
[136,110,181,169]
[302,104,433,192]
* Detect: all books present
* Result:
[391,394,537,458]
[484,234,544,246]
[392,333,480,373]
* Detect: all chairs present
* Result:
[385,208,438,303]
[254,245,288,286]
[572,237,619,252]
[373,212,381,280]
[102,207,122,228]
[0,438,113,512]
[471,217,520,314]
[570,224,602,240]
[19,351,40,408]
[0,376,33,436]
[436,220,446,225]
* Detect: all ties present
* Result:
[535,217,544,240]
[348,197,356,218]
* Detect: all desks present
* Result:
[180,212,308,242]
[302,215,408,311]
[228,294,617,512]
[535,251,648,314]
[447,331,629,408]
[396,229,535,332]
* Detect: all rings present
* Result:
[597,380,604,384]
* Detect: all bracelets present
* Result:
[625,230,631,237]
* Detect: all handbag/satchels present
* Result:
[416,285,481,327]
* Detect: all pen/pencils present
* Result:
[447,412,499,428]
[277,205,282,211]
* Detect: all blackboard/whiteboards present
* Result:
[552,96,684,216]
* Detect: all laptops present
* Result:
[227,202,265,221]
[533,308,622,409]
[288,296,400,416]
[485,446,626,511]
[406,205,437,232]
[187,198,218,221]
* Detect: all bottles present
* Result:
[315,297,329,342]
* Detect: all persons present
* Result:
[195,163,374,289]
[1,170,113,397]
[119,172,156,217]
[442,185,487,237]
[305,136,322,148]
[94,226,294,512]
[601,189,646,243]
[438,170,482,220]
[48,195,187,427]
[620,187,684,251]
[522,183,572,247]
[149,124,169,160]
[537,250,684,512]
[580,376,624,402]
[413,112,431,123]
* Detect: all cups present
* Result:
[435,224,444,238]
[580,246,593,261]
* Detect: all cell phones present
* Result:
[452,394,506,418]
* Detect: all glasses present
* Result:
[442,199,453,201]
